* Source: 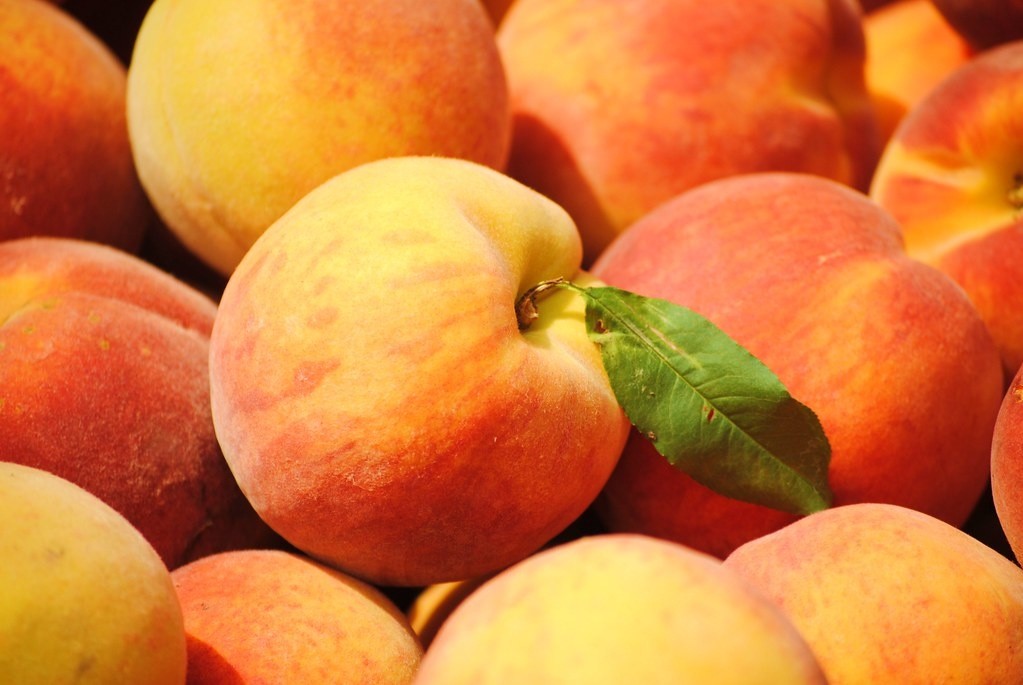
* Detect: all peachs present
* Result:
[0,0,1023,685]
